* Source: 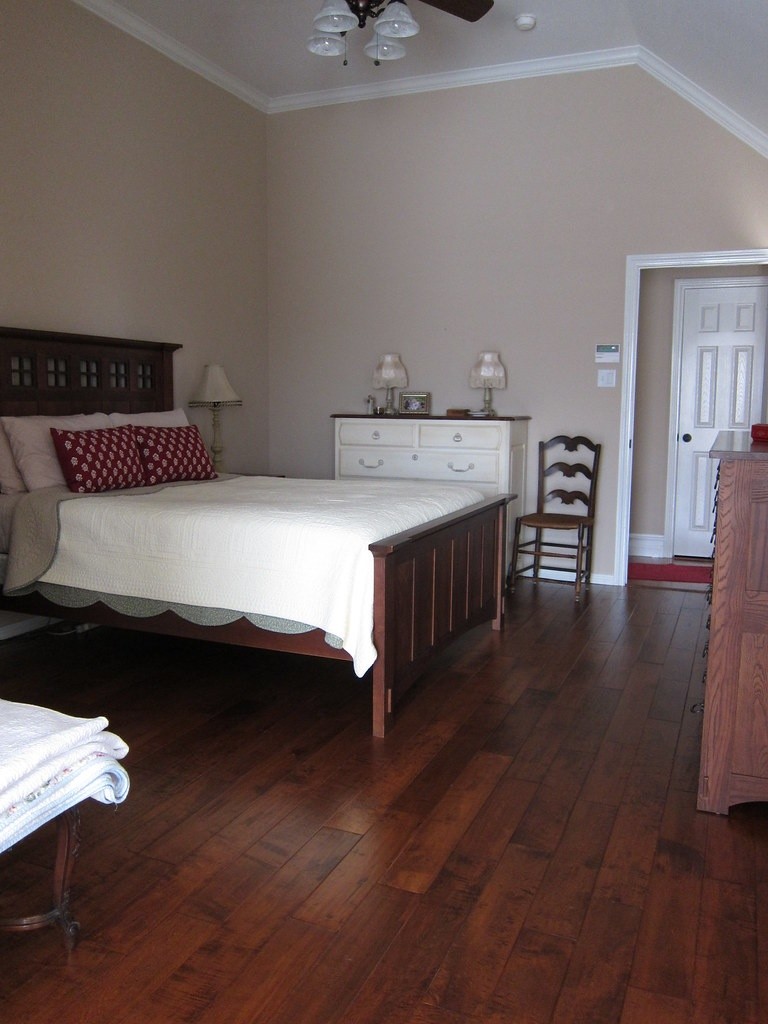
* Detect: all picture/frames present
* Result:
[399,392,430,414]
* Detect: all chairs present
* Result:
[510,436,601,602]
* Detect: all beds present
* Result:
[0,326,518,738]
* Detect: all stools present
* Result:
[1,808,81,952]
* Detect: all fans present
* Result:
[340,0,494,65]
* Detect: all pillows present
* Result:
[1,413,114,492]
[135,424,219,486]
[0,422,28,494]
[49,423,147,493]
[109,408,190,428]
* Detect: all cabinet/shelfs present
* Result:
[330,413,531,590]
[689,431,768,812]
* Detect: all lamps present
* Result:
[470,352,505,417]
[372,353,407,415]
[305,0,421,61]
[188,362,243,473]
